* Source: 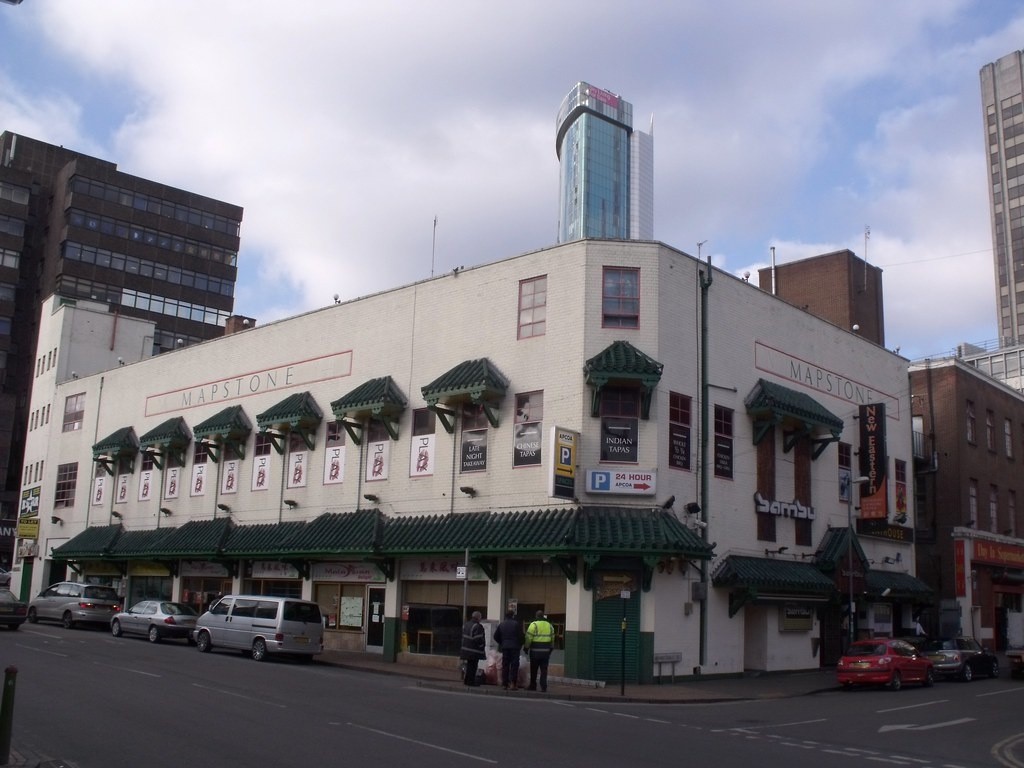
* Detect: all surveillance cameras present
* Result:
[693,519,708,529]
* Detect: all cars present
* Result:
[0,568,12,586]
[110,599,201,643]
[837,639,935,691]
[922,636,1000,685]
[1005,637,1024,679]
[0,589,28,631]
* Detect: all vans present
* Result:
[403,606,465,653]
[194,594,325,662]
[28,581,121,630]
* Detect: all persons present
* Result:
[460,611,486,687]
[523,610,556,692]
[494,611,524,690]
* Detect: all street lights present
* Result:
[847,476,871,641]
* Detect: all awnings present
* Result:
[728,588,830,618]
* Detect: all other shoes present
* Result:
[464,682,480,687]
[503,685,508,689]
[542,688,547,692]
[510,682,518,690]
[524,686,537,691]
